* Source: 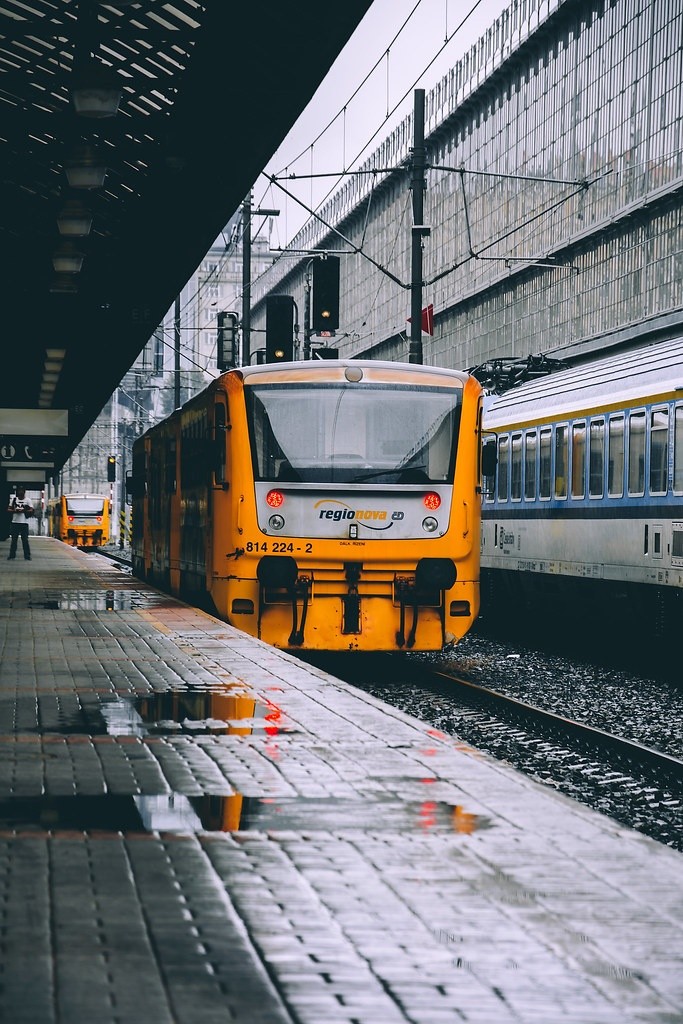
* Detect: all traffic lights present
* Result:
[313,255,339,330]
[265,293,294,363]
[107,455,116,482]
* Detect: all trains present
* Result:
[48,493,114,550]
[482,335,683,588]
[129,359,500,651]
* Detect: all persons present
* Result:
[7,485,35,561]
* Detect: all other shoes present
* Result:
[25,556,32,561]
[7,554,16,560]
